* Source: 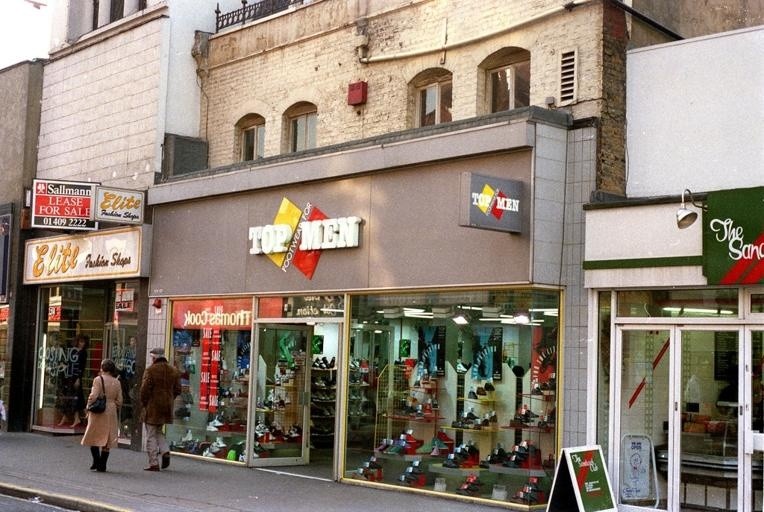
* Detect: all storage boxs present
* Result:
[682,413,725,432]
[38,407,63,427]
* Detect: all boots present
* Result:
[90,446,109,472]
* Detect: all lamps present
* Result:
[677,188,708,230]
[512,310,531,325]
[451,305,473,325]
[662,304,735,315]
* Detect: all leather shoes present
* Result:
[144,465,159,471]
[161,452,170,469]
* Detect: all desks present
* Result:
[666,430,737,455]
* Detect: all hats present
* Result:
[151,348,165,358]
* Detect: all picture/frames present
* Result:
[0,202,14,304]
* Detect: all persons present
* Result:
[79,357,124,473]
[138,347,182,471]
[116,333,137,441]
[55,335,90,428]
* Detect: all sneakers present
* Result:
[251,421,301,440]
[312,356,554,506]
[169,438,259,461]
[257,396,285,410]
[513,478,543,503]
[175,393,193,416]
[479,441,536,468]
[205,415,243,433]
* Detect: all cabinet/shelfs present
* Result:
[310,362,378,437]
[372,373,557,479]
[172,351,306,448]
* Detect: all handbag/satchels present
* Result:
[87,375,106,413]
